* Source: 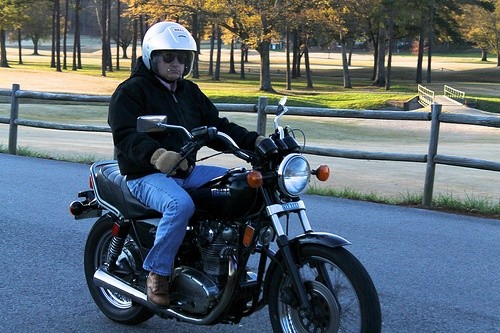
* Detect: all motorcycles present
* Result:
[68,95,383,333]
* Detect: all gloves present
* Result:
[150,148,188,175]
[256,135,269,147]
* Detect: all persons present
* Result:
[107,21,273,307]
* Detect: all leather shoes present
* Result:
[145,271,170,309]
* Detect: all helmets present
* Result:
[141,22,198,81]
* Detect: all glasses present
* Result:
[153,51,188,65]
